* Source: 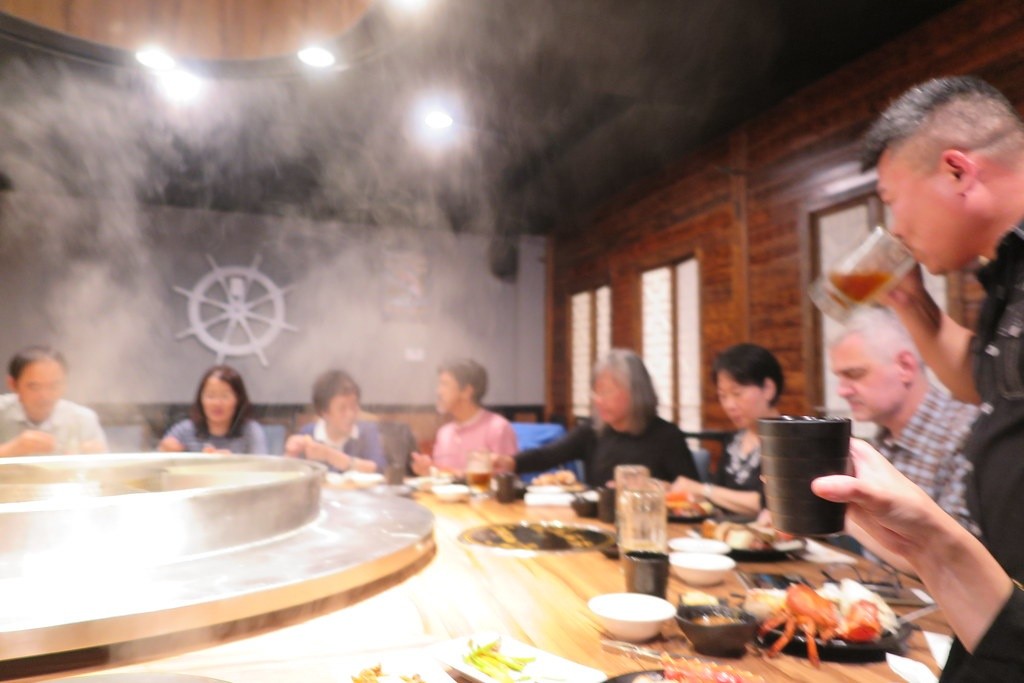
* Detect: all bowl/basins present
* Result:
[401,474,451,492]
[346,473,384,490]
[586,592,677,643]
[431,484,470,502]
[667,551,736,588]
[568,497,599,518]
[667,536,732,558]
[674,603,756,657]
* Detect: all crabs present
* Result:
[702,520,783,550]
[761,582,841,670]
[666,491,705,518]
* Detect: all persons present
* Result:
[155,362,269,455]
[409,357,519,482]
[0,343,109,458]
[830,310,993,588]
[282,368,384,476]
[852,76,1023,586]
[794,415,1023,682]
[605,343,785,518]
[475,349,700,490]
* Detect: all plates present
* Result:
[664,497,754,524]
[733,567,814,592]
[373,484,415,497]
[274,630,608,683]
[737,598,910,649]
[34,670,232,683]
[524,484,565,493]
[596,668,665,683]
[691,526,807,562]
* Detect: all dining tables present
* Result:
[0,480,954,683]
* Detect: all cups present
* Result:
[614,465,668,565]
[596,485,616,523]
[467,463,491,501]
[807,225,917,327]
[753,414,852,536]
[495,472,515,503]
[622,550,670,604]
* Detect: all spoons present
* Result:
[437,660,486,683]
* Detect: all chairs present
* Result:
[677,424,736,486]
[263,423,290,455]
[510,417,568,480]
[377,420,420,467]
